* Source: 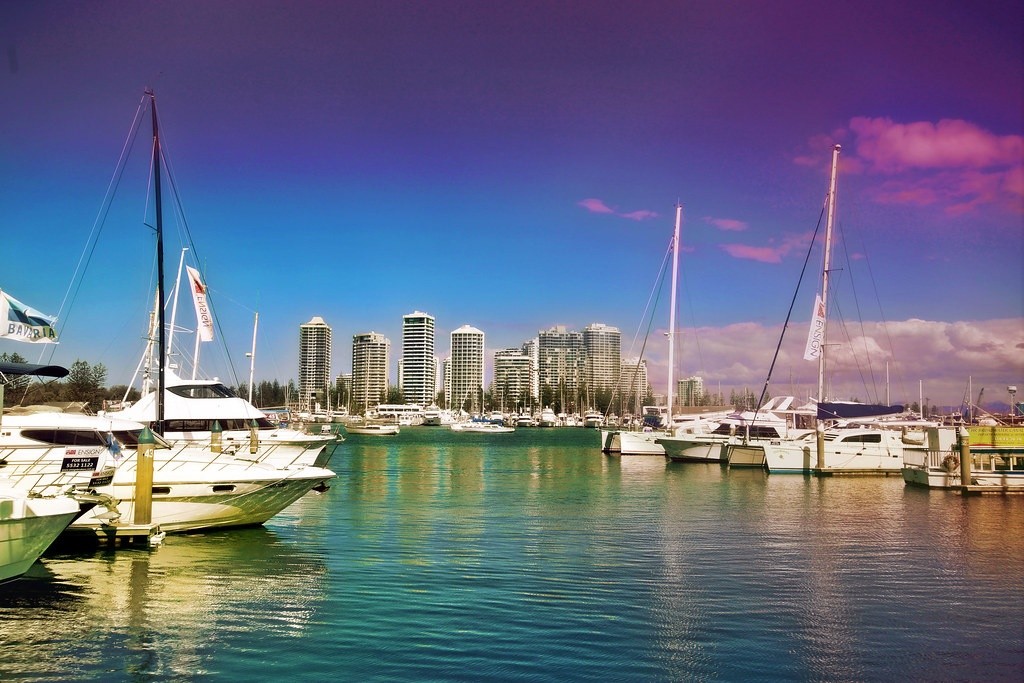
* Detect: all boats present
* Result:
[289,402,604,435]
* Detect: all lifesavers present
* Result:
[943,454,960,472]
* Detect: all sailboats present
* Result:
[599,142,1024,489]
[0,83,347,588]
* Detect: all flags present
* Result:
[0,289,59,345]
[185,265,214,341]
[803,292,826,360]
